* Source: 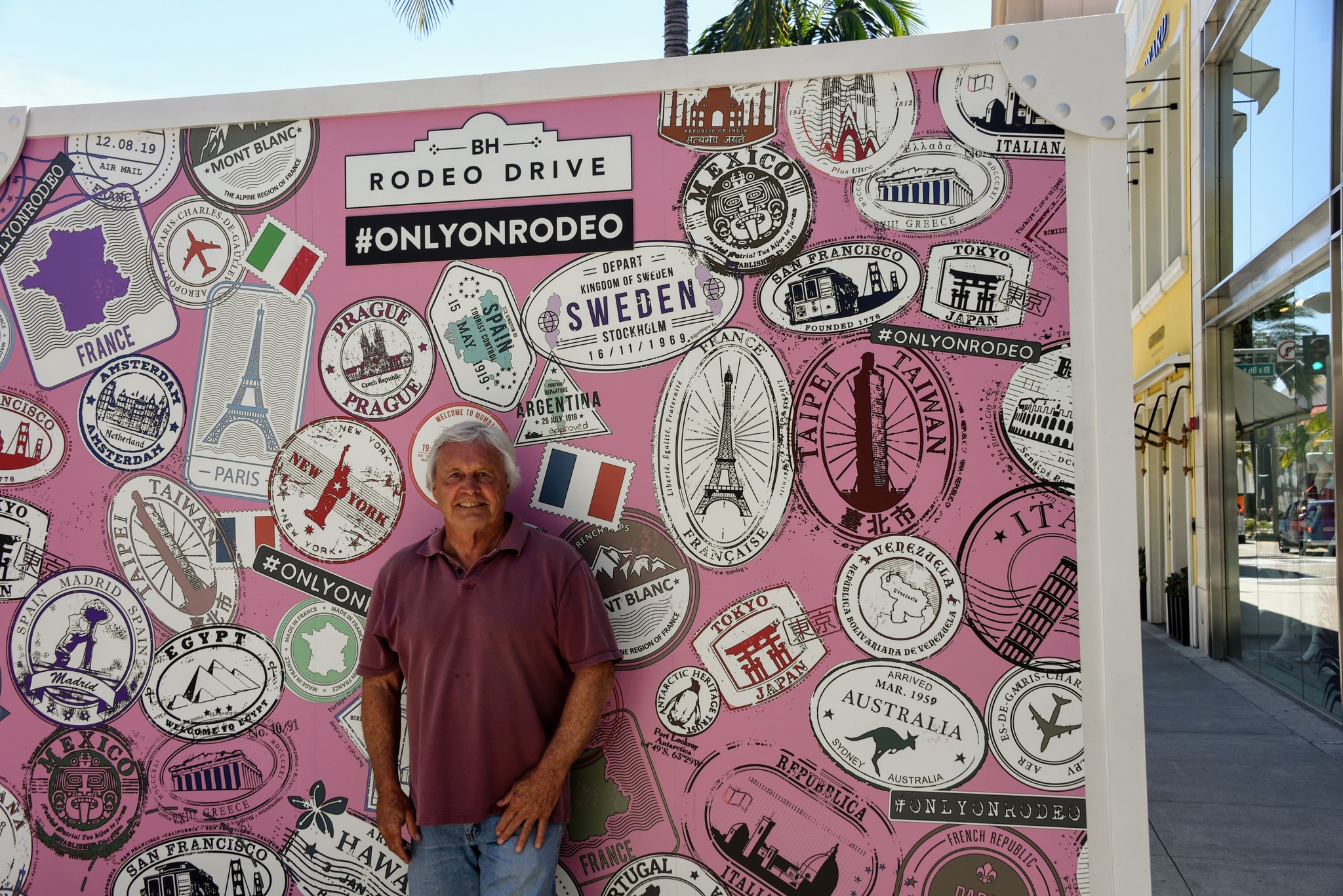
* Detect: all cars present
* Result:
[1237,504,1245,544]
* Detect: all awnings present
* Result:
[1233,365,1311,442]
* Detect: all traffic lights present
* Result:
[1303,335,1330,375]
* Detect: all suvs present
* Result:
[1277,499,1335,553]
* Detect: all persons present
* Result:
[357,421,623,896]
[1269,438,1335,664]
